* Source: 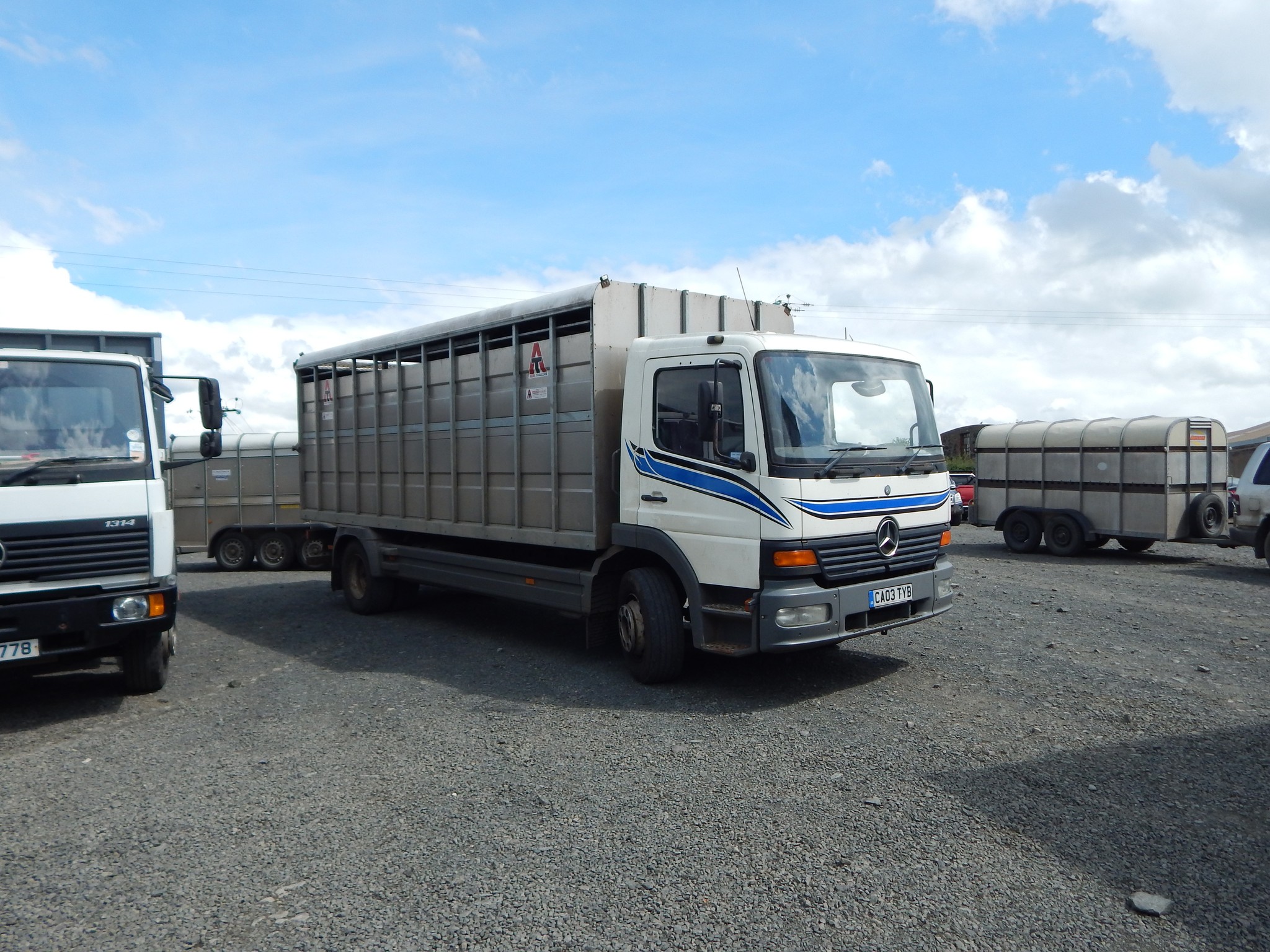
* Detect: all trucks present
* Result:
[968,415,1270,571]
[292,276,961,690]
[0,328,224,698]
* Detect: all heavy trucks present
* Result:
[166,431,337,572]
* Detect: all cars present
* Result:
[1226,477,1241,516]
[947,470,975,526]
